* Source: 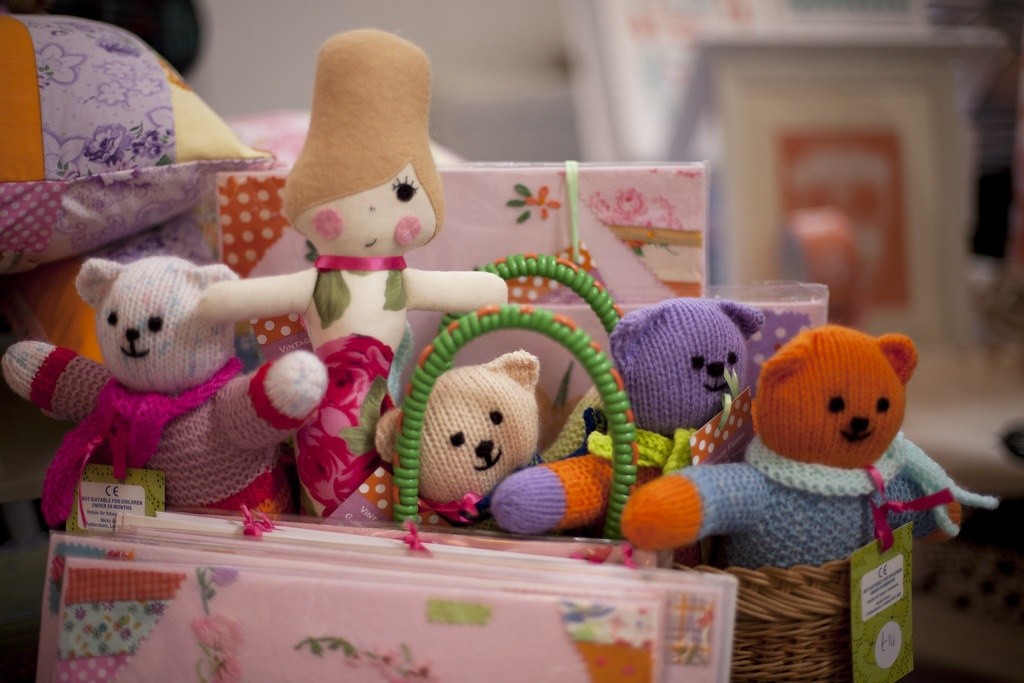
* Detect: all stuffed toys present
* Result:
[1,25,1002,572]
